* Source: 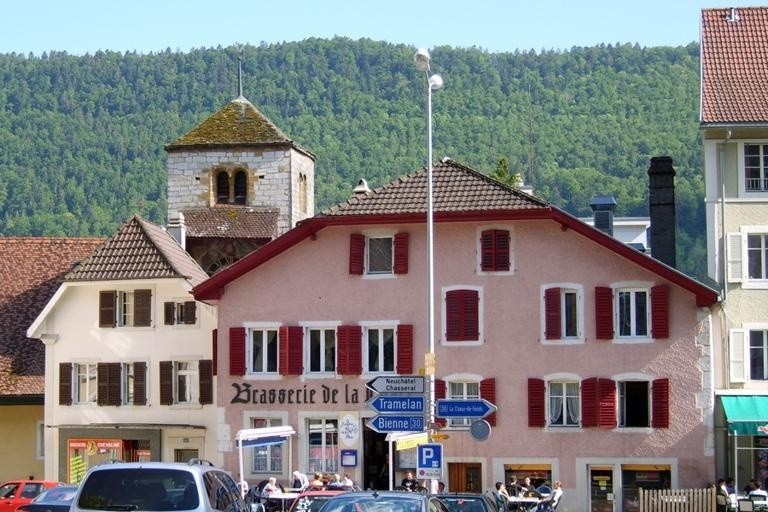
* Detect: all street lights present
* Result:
[405,39,450,497]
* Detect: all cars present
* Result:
[591,496,639,512]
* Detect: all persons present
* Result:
[439,482,445,492]
[379,454,389,490]
[494,475,563,512]
[402,470,418,489]
[291,470,353,493]
[707,477,768,512]
[260,477,280,512]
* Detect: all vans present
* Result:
[66,456,248,512]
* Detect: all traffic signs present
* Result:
[363,414,425,435]
[363,375,424,394]
[364,395,426,414]
[429,433,450,441]
[435,399,497,420]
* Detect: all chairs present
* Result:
[245,478,428,511]
[490,483,562,512]
